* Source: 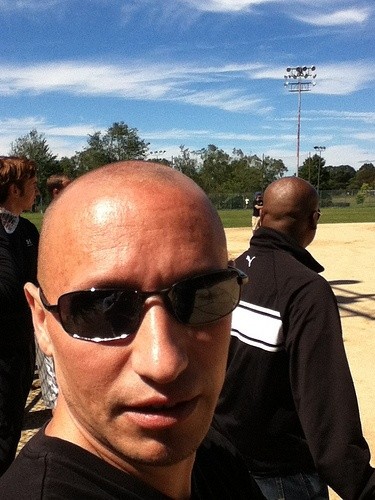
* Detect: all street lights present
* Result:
[313,146,326,196]
[283,65,317,179]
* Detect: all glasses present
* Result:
[314,208,321,221]
[258,197,262,200]
[39,265,248,343]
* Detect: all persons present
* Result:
[252,193,263,234]
[245,197,250,209]
[211,177,375,500]
[0,155,41,478]
[0,160,268,500]
[34,173,71,409]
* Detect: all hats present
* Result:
[256,192,261,195]
